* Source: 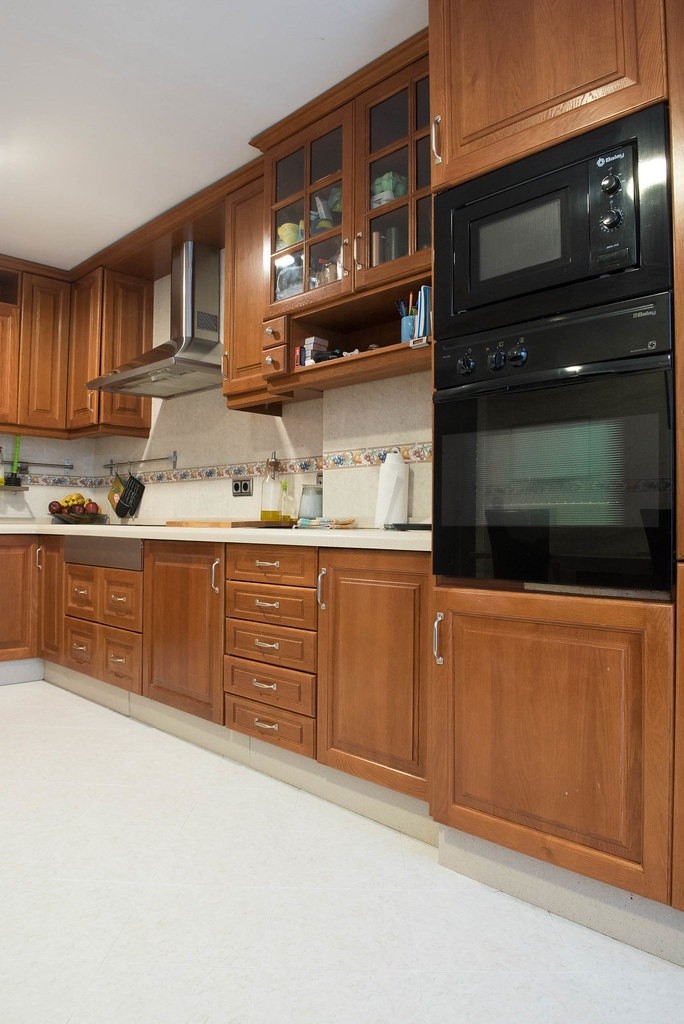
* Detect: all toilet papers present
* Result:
[374,453,410,529]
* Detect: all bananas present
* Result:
[60,493,92,507]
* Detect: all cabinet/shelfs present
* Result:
[0,535,684,911]
[222,0,669,418]
[0,268,150,439]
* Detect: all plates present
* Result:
[47,514,108,524]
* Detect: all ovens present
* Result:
[431,99,677,603]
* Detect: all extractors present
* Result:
[84,240,224,401]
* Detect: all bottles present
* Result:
[371,231,383,267]
[0,447,5,485]
[298,484,323,520]
[17,470,30,485]
[261,458,282,521]
[387,226,399,262]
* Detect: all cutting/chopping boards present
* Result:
[165,521,262,528]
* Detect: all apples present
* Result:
[48,501,98,514]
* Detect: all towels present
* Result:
[293,515,359,531]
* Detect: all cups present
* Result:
[402,315,415,344]
